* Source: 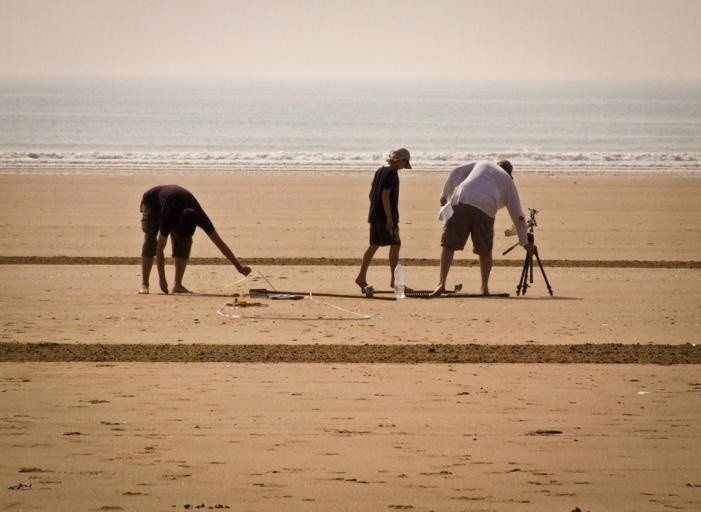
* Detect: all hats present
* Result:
[496,160,513,179]
[386,148,412,169]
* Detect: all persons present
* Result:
[429,159,537,296]
[136,185,251,294]
[355,147,414,294]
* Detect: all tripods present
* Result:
[516,240,553,297]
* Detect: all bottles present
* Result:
[394,261,405,299]
